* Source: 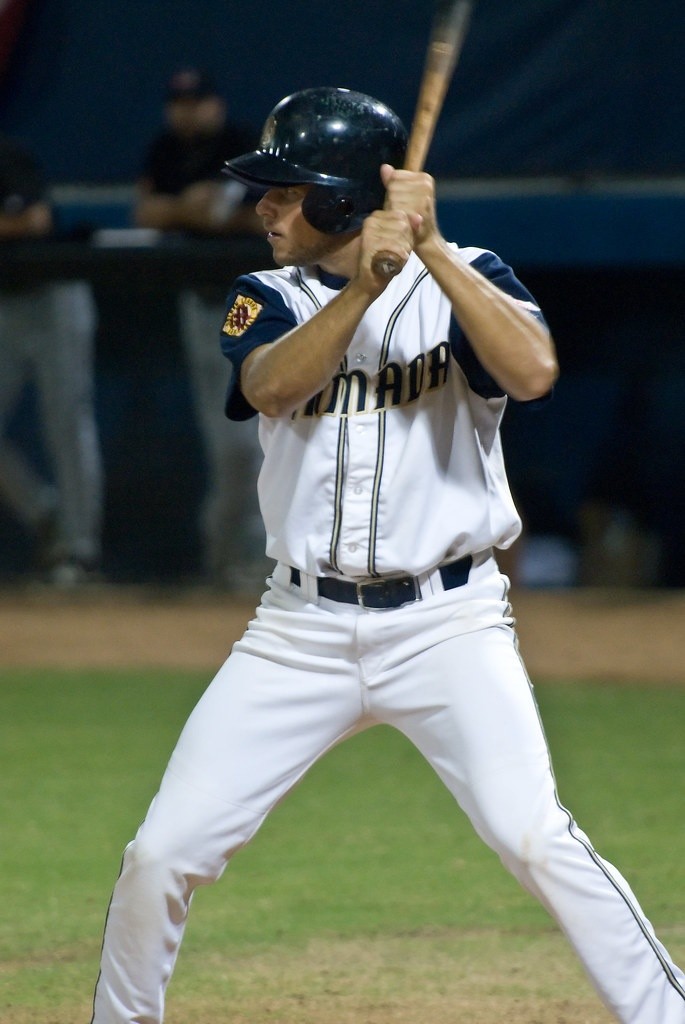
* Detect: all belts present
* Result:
[291,555,473,609]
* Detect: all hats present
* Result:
[163,72,210,100]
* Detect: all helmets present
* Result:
[223,87,409,235]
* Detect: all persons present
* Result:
[87,89,685,1024]
[0,71,279,597]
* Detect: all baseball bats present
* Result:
[371,0,476,276]
